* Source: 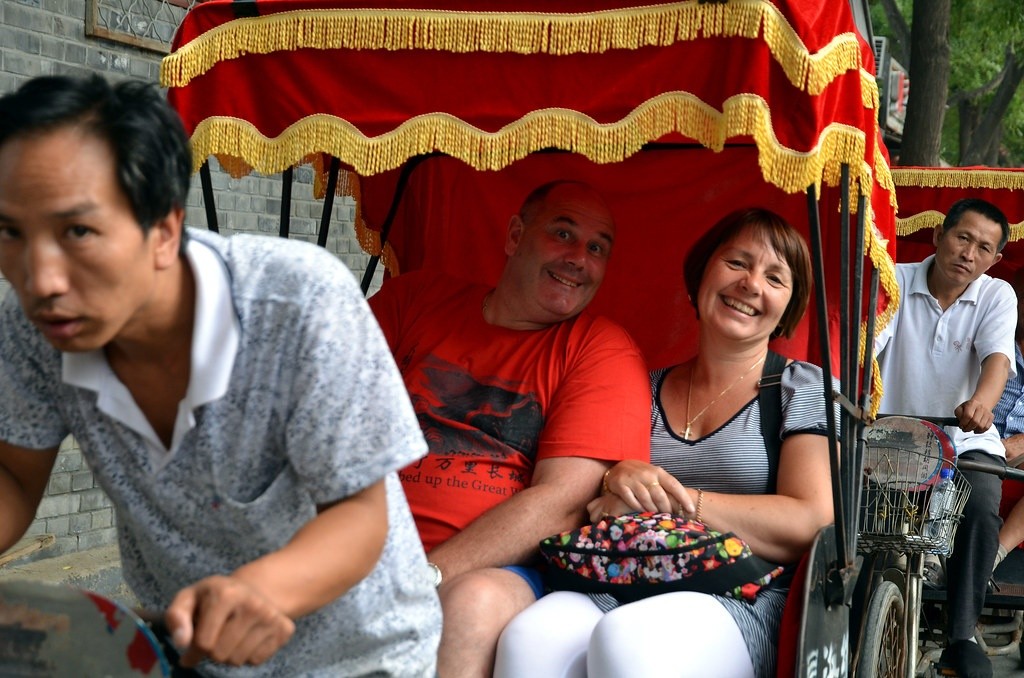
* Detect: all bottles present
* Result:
[928,467,957,545]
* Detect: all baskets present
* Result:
[857,445,972,559]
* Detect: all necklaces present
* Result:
[680,351,768,440]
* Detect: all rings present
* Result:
[647,482,659,489]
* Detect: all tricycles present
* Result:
[854,165,1024,678]
[0,0,897,678]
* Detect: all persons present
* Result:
[0,74,443,678]
[922,270,1024,593]
[494,207,840,677]
[367,179,651,678]
[874,196,1018,678]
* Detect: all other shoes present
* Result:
[938,640,993,678]
[986,573,1000,594]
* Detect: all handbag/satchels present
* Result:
[540,513,785,604]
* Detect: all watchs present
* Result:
[428,563,442,590]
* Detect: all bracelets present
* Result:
[603,469,612,496]
[697,489,703,522]
[679,503,685,517]
